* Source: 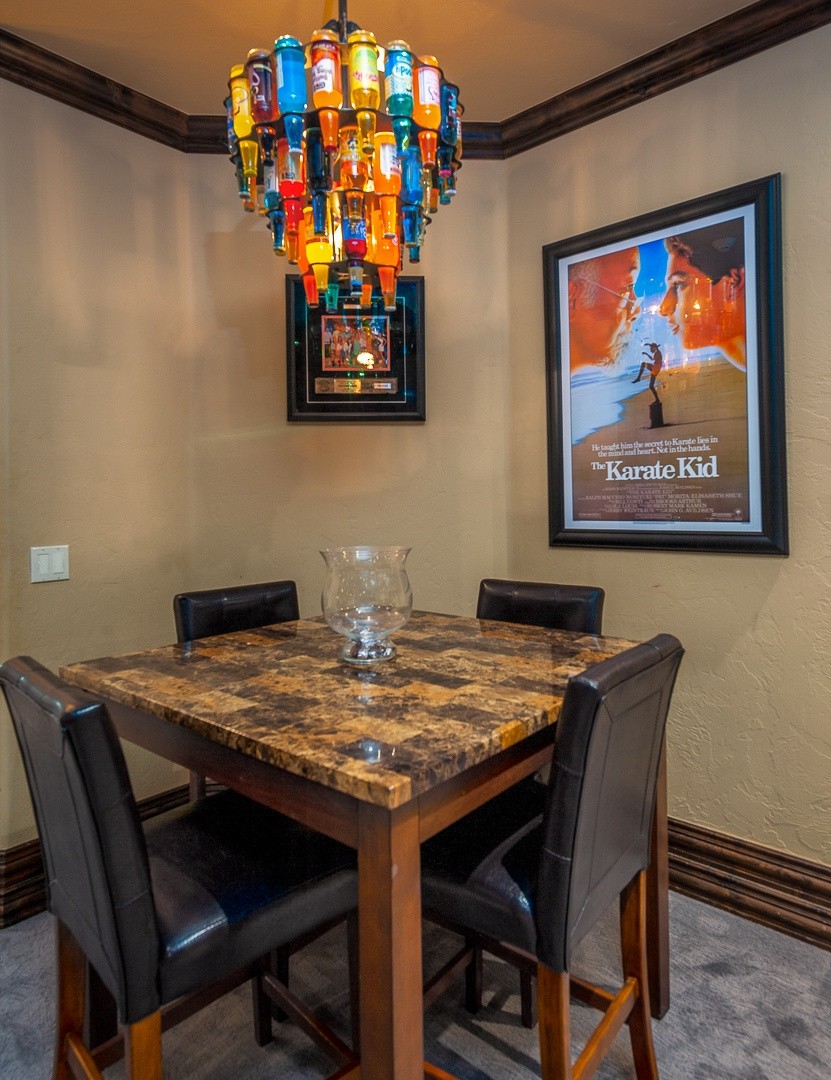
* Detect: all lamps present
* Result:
[221,0,466,314]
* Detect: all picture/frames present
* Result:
[284,273,427,423]
[541,173,791,559]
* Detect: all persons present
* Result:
[563,246,643,381]
[629,342,664,404]
[324,316,388,370]
[655,216,749,373]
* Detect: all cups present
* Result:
[318,544,415,666]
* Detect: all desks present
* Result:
[58,602,652,1080]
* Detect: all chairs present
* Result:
[172,578,304,804]
[476,579,605,637]
[0,655,360,1080]
[421,632,685,1080]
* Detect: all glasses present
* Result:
[578,274,645,315]
